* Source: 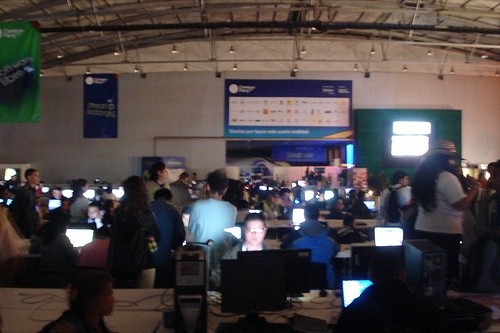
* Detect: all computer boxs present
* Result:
[172,243,210,333]
[403,239,447,303]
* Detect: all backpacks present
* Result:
[385,186,404,223]
[336,226,368,244]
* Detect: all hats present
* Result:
[420,139,465,160]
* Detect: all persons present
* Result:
[0,139,500,333]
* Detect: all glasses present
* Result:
[247,227,264,234]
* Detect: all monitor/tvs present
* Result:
[41,187,125,212]
[345,188,352,198]
[221,259,286,323]
[65,227,95,249]
[351,246,404,281]
[373,226,404,246]
[341,278,376,308]
[238,248,311,295]
[363,201,377,213]
[292,207,306,230]
[305,190,315,201]
[324,190,334,201]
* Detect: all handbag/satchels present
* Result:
[436,298,492,333]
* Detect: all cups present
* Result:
[162,308,174,327]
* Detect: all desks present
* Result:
[0,218,500,333]
